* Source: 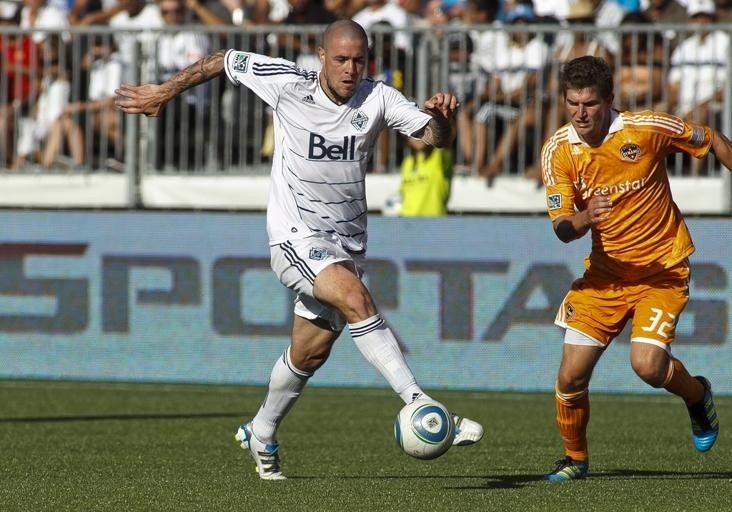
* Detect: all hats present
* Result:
[687,0,716,18]
[566,1,596,24]
[505,5,535,24]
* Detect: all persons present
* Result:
[1,0,731,217]
[115,21,483,481]
[542,56,732,481]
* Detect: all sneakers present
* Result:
[544,457,590,481]
[235,420,287,480]
[689,376,720,452]
[450,414,484,447]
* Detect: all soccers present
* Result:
[394,398,455,460]
